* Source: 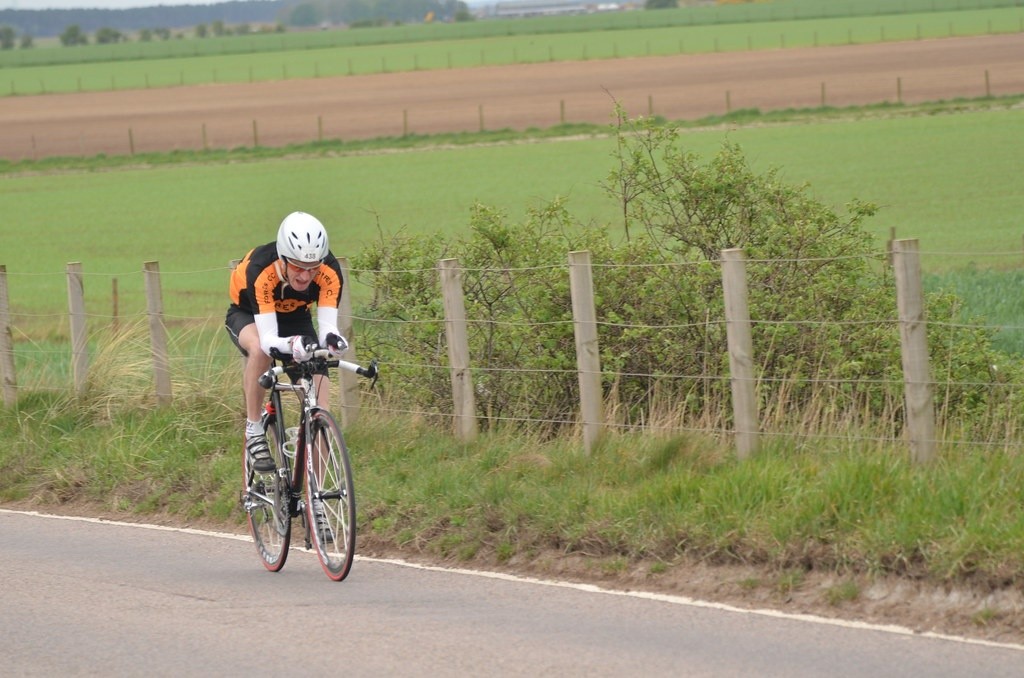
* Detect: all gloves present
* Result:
[325,332,349,358]
[290,334,318,365]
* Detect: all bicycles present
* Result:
[240,340,378,584]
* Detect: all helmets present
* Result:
[275,212,329,263]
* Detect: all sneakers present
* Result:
[243,430,276,471]
[315,509,334,543]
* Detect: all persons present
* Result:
[224,211,350,544]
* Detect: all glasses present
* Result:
[286,262,321,272]
